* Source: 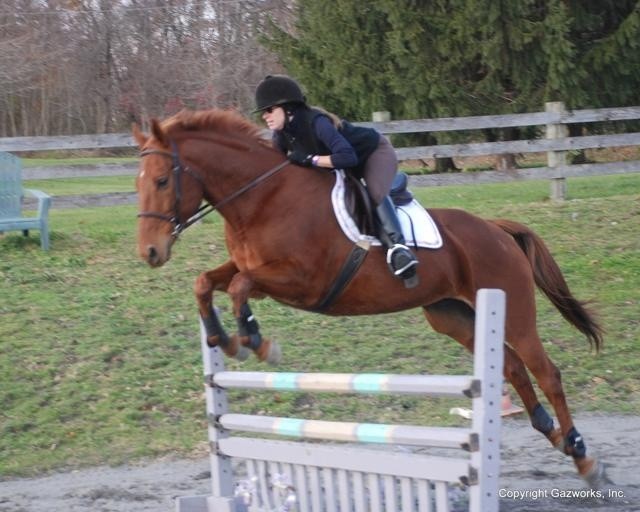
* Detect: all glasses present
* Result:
[266,106,280,113]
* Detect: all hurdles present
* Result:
[200,289,505,512]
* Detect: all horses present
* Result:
[130,107,610,494]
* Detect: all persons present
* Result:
[250,75,421,290]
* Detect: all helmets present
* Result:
[252,75,304,112]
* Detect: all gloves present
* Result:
[286,149,319,166]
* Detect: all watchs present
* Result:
[311,155,320,166]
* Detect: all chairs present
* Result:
[0,152,53,251]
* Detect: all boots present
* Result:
[373,195,416,278]
